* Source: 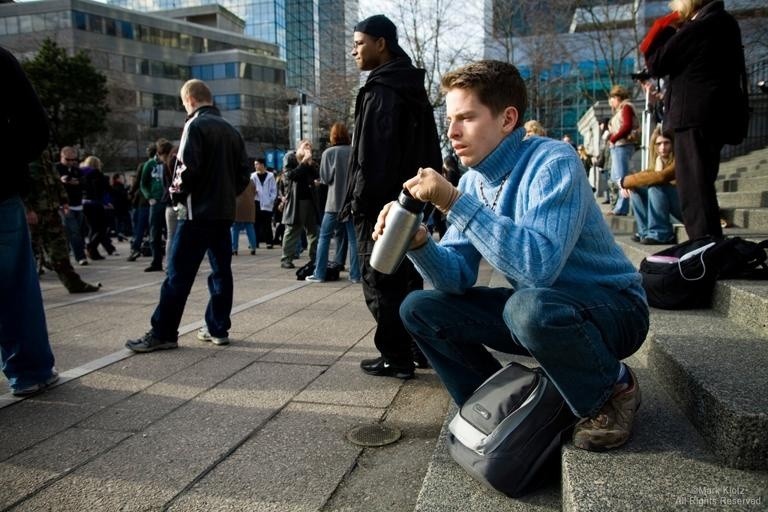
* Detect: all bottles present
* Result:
[369,186,426,276]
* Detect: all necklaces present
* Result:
[477,178,504,213]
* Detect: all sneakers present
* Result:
[69,283,98,293]
[280,262,295,268]
[11,366,59,396]
[602,200,627,215]
[631,233,677,244]
[348,275,360,283]
[197,325,230,345]
[305,275,324,283]
[127,249,162,272]
[572,362,642,452]
[124,331,178,353]
[248,244,273,255]
[360,354,429,379]
[78,250,120,265]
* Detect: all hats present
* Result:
[355,14,408,58]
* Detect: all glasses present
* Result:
[65,158,75,161]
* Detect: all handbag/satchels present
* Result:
[724,90,748,144]
[447,361,581,499]
[296,260,339,281]
[638,235,767,310]
[625,127,641,141]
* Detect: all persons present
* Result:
[372,59,650,452]
[231,122,360,284]
[563,64,685,245]
[25,120,177,294]
[124,76,250,354]
[645,0,755,240]
[444,155,462,187]
[350,13,442,380]
[524,119,545,138]
[1,45,61,396]
